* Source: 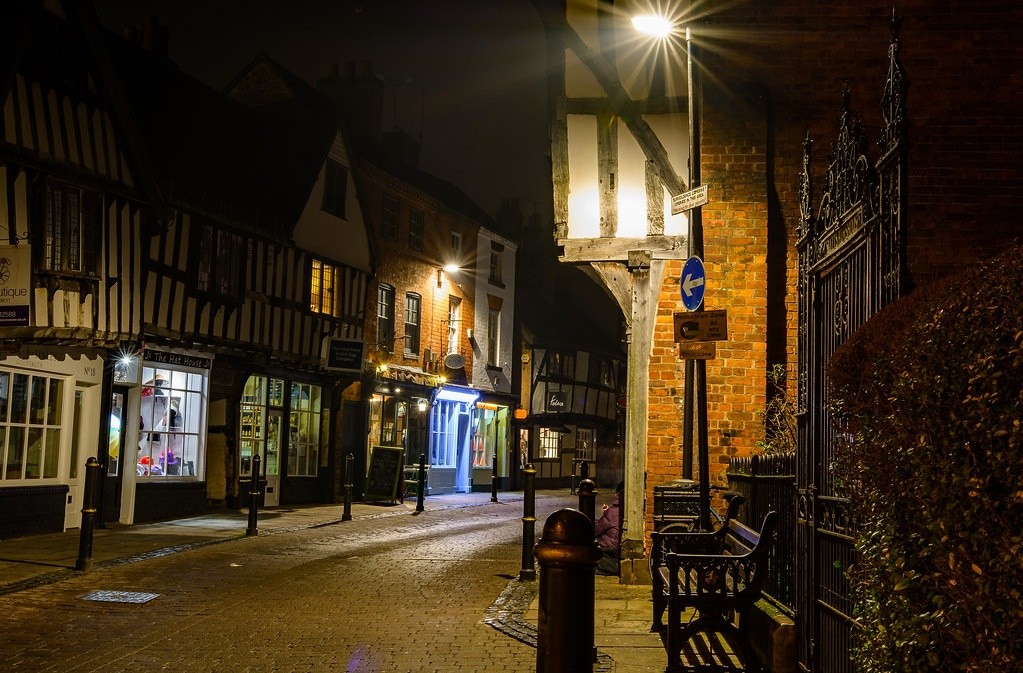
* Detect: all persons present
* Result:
[594,480,624,562]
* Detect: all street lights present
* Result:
[627,11,693,480]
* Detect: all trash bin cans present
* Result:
[653,478,702,564]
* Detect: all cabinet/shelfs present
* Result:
[240,409,261,440]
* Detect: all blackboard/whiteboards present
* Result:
[364,445,402,500]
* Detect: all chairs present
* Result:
[403,464,431,501]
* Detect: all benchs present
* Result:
[650,495,780,673]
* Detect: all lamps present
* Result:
[417,398,429,412]
